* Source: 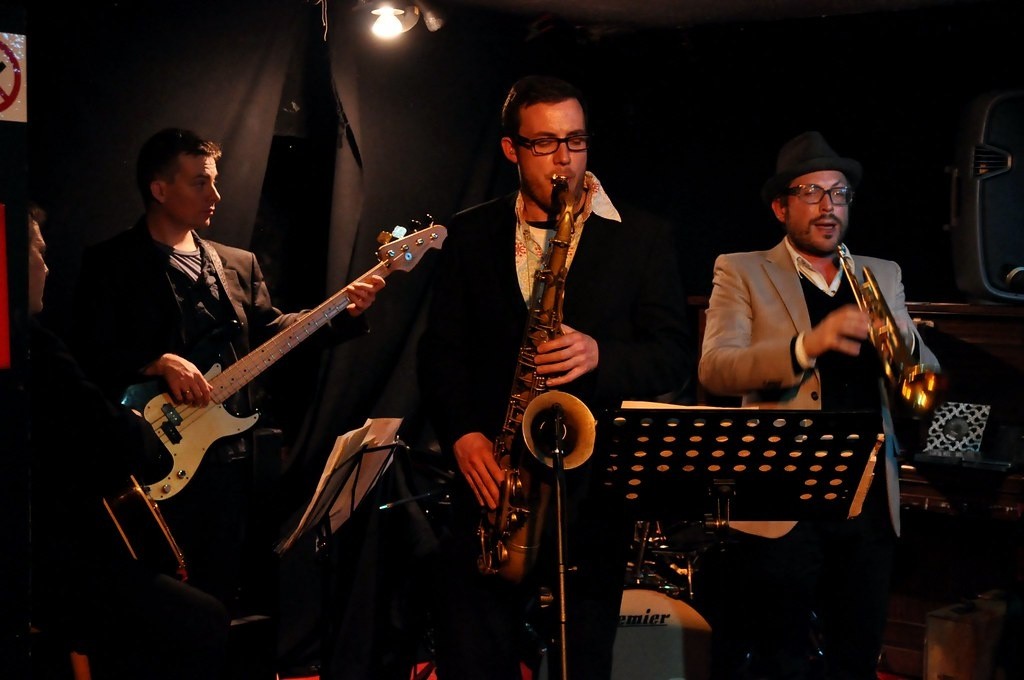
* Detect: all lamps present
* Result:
[367,0,404,40]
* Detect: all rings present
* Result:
[183,390,192,396]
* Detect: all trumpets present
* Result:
[834,245,943,412]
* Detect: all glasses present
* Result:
[781,183,856,206]
[515,130,597,156]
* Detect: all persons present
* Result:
[698,131,943,680]
[419,72,697,680]
[26,205,51,316]
[69,127,386,628]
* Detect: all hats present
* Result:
[761,130,863,200]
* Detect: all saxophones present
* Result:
[463,175,597,590]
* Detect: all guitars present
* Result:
[99,213,449,576]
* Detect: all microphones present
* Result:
[550,404,565,478]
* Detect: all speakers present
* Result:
[940,86,1024,304]
